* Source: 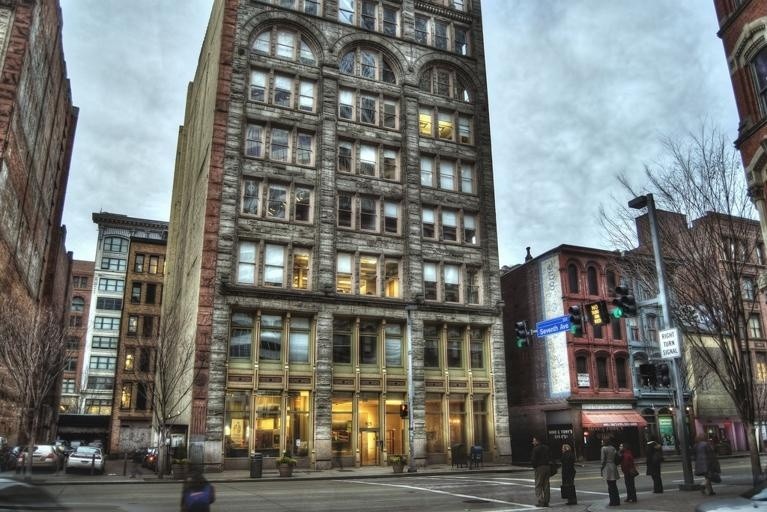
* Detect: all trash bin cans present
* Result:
[250,453,263,478]
[451,444,483,468]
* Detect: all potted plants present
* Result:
[388,455,408,473]
[274,456,297,477]
[172,459,191,478]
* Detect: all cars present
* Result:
[134,447,159,472]
[694,480,767,512]
[0,437,108,475]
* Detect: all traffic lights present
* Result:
[612,286,636,318]
[513,320,527,348]
[640,365,658,387]
[568,306,580,338]
[400,404,408,417]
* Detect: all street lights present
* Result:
[628,193,694,486]
[404,303,418,473]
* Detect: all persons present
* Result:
[643,431,663,492]
[182,462,215,511]
[559,443,578,506]
[331,437,343,470]
[129,446,143,479]
[530,433,553,507]
[690,431,715,495]
[617,441,638,502]
[598,437,620,506]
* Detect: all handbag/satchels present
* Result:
[559,482,576,500]
[183,484,215,509]
[627,465,640,479]
